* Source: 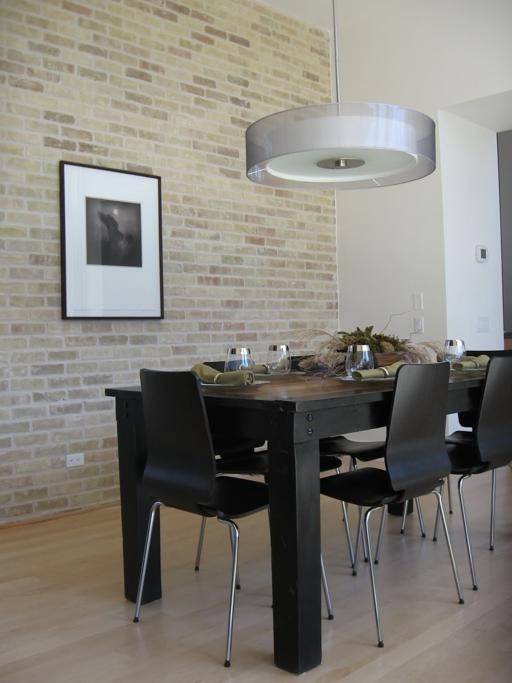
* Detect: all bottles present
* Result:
[223,346,256,371]
[443,339,466,363]
[265,345,291,376]
[345,344,375,376]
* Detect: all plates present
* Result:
[335,376,395,384]
[199,380,271,392]
[450,368,487,374]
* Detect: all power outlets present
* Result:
[65,453,85,467]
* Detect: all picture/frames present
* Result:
[59,158,166,319]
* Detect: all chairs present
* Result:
[445,350,512,514]
[133,366,332,667]
[320,363,464,649]
[290,355,387,564]
[400,357,510,592]
[194,359,358,578]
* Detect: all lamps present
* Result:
[246,0,436,189]
